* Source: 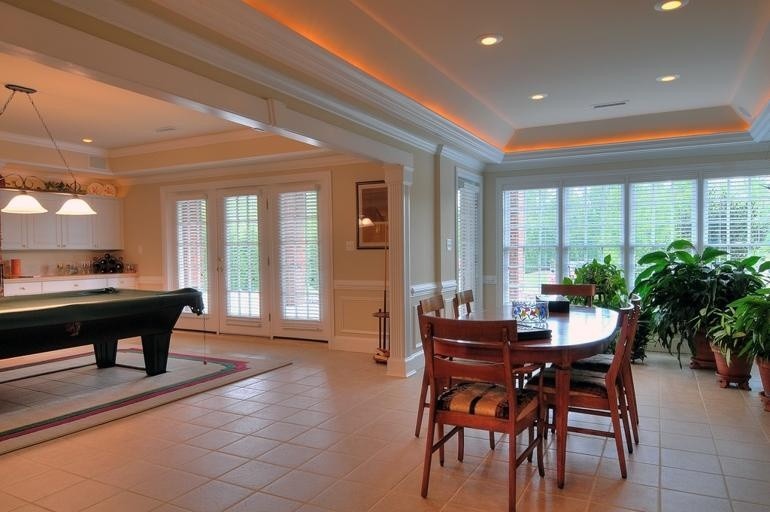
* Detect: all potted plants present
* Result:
[629,240,769,412]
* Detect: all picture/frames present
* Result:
[354,180,388,249]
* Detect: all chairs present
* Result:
[415,284,639,510]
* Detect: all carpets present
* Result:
[0,345,295,457]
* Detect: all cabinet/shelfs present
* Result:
[0,188,125,252]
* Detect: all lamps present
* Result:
[1,84,98,216]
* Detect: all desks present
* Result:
[0,287,205,377]
